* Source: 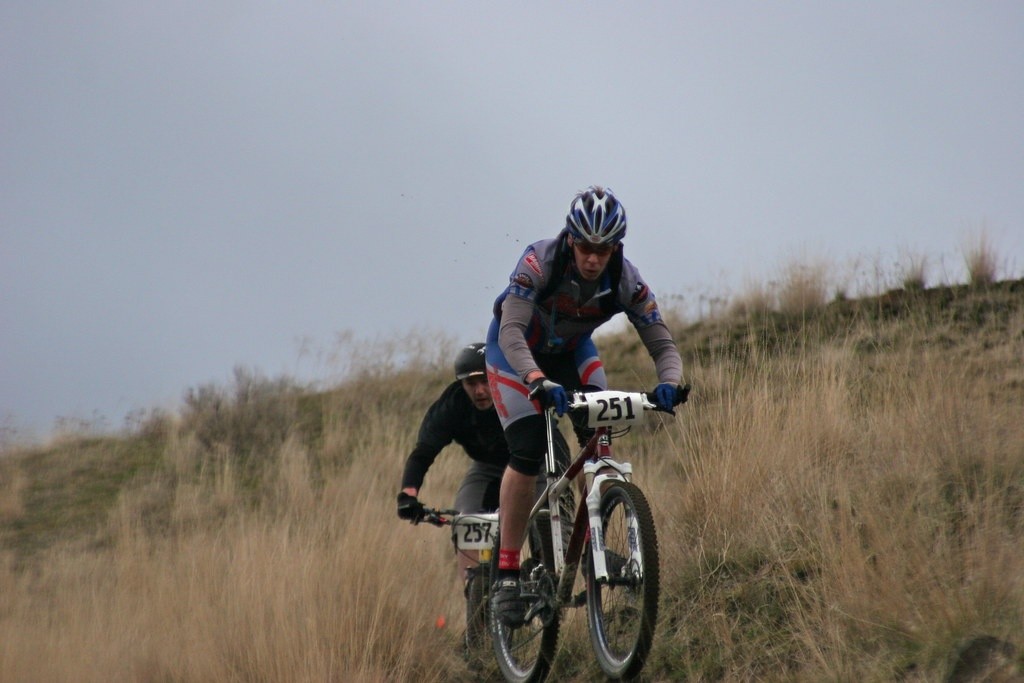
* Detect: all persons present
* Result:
[492,188,686,627]
[397,343,571,647]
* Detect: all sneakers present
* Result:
[581,548,628,580]
[492,577,524,629]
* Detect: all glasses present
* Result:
[572,235,615,257]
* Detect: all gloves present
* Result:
[654,382,683,411]
[396,492,425,525]
[530,377,569,417]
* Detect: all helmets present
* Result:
[566,188,626,245]
[455,343,486,380]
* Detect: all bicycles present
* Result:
[406,500,519,671]
[485,382,693,682]
